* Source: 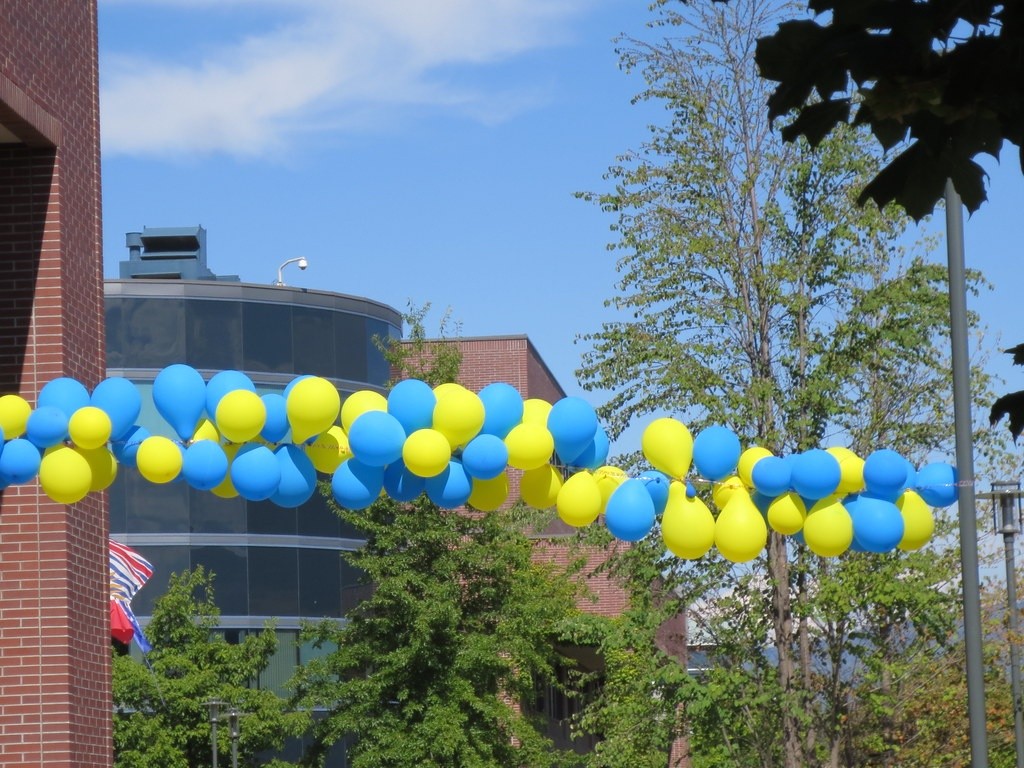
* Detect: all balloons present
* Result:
[0,359,957,564]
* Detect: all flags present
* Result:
[108,539,153,648]
